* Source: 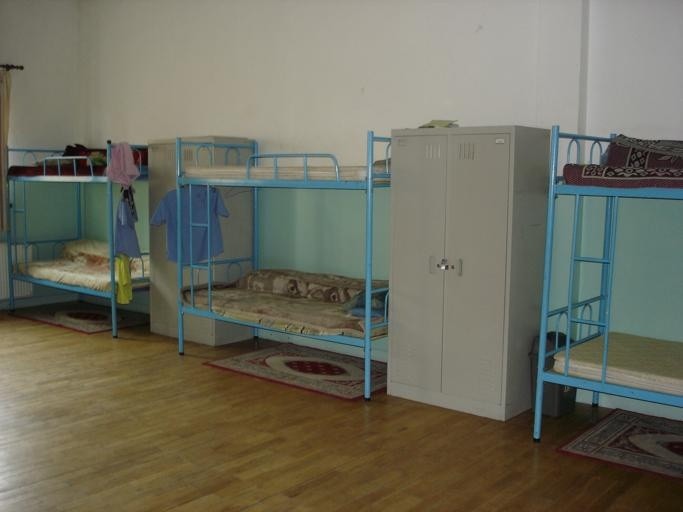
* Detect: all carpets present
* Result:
[202,342,387,401]
[7,299,150,335]
[553,407,683,484]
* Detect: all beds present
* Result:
[175,129,392,401]
[6,138,148,338]
[532,124,683,445]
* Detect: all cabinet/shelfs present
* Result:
[386,125,551,424]
[148,134,254,348]
